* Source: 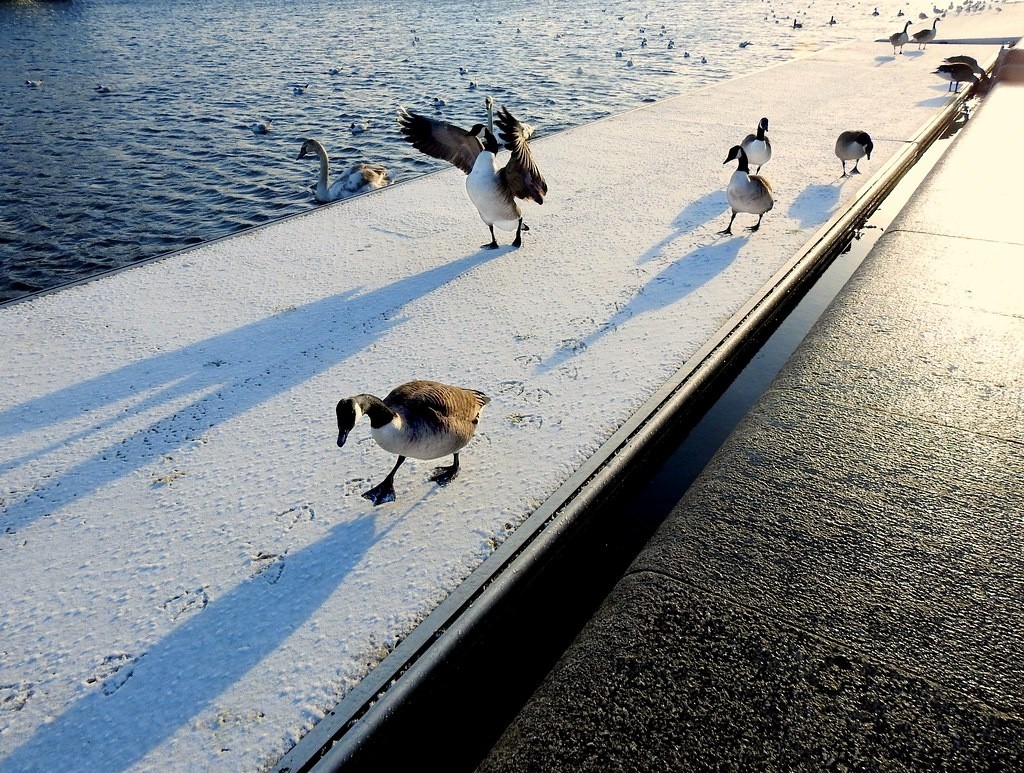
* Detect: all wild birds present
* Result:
[888,20,913,55]
[584,8,649,24]
[411,29,421,45]
[638,28,644,33]
[329,67,343,76]
[667,40,674,49]
[684,51,690,57]
[335,380,492,508]
[941,54,991,86]
[97,84,114,93]
[475,16,524,33]
[829,16,836,25]
[349,120,373,133]
[717,145,774,236]
[905,0,1009,20]
[615,49,624,58]
[912,17,942,51]
[469,81,478,88]
[292,83,310,96]
[739,40,751,48]
[458,67,468,75]
[872,8,880,16]
[929,64,984,97]
[395,94,548,251]
[796,1,815,16]
[659,25,667,37]
[834,130,874,179]
[433,97,447,106]
[701,56,708,63]
[761,0,790,24]
[26,79,44,86]
[793,19,803,29]
[739,117,772,175]
[897,9,905,17]
[836,1,861,8]
[640,37,647,47]
[249,118,272,133]
